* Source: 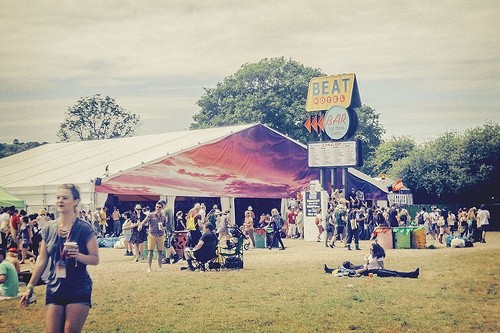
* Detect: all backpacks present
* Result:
[315,218,319,225]
[377,213,385,224]
[186,214,198,231]
[418,211,426,224]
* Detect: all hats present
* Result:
[135,204,142,209]
[248,206,252,210]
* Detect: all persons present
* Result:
[243,212,255,246]
[158,200,182,263]
[0,250,19,297]
[286,198,304,239]
[326,186,412,251]
[138,202,166,272]
[185,202,223,248]
[324,239,419,279]
[415,204,491,247]
[20,183,100,333]
[184,222,218,271]
[0,206,55,264]
[259,208,285,250]
[176,211,186,231]
[245,206,255,219]
[315,209,324,242]
[79,204,151,262]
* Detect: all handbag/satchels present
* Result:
[327,224,334,232]
[350,219,358,230]
[446,236,473,248]
[337,225,343,234]
[221,257,243,269]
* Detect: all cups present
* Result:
[158,222,163,230]
[369,272,377,278]
[64,241,78,269]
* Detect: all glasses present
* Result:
[157,206,161,208]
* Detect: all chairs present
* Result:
[220,237,245,267]
[192,240,219,271]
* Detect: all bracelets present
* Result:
[27,284,33,290]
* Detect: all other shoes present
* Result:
[134,259,139,262]
[147,269,151,272]
[162,258,170,264]
[128,251,133,256]
[324,264,331,273]
[267,235,362,250]
[35,255,37,262]
[19,260,25,264]
[172,255,182,264]
[183,266,194,271]
[159,268,163,271]
[413,268,419,278]
[124,251,129,255]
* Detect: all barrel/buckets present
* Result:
[266,228,279,248]
[374,227,427,250]
[253,228,265,248]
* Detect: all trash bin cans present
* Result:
[266,228,279,248]
[174,231,188,257]
[392,227,410,250]
[373,227,393,250]
[410,226,426,249]
[252,228,265,248]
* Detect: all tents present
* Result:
[0,122,394,227]
